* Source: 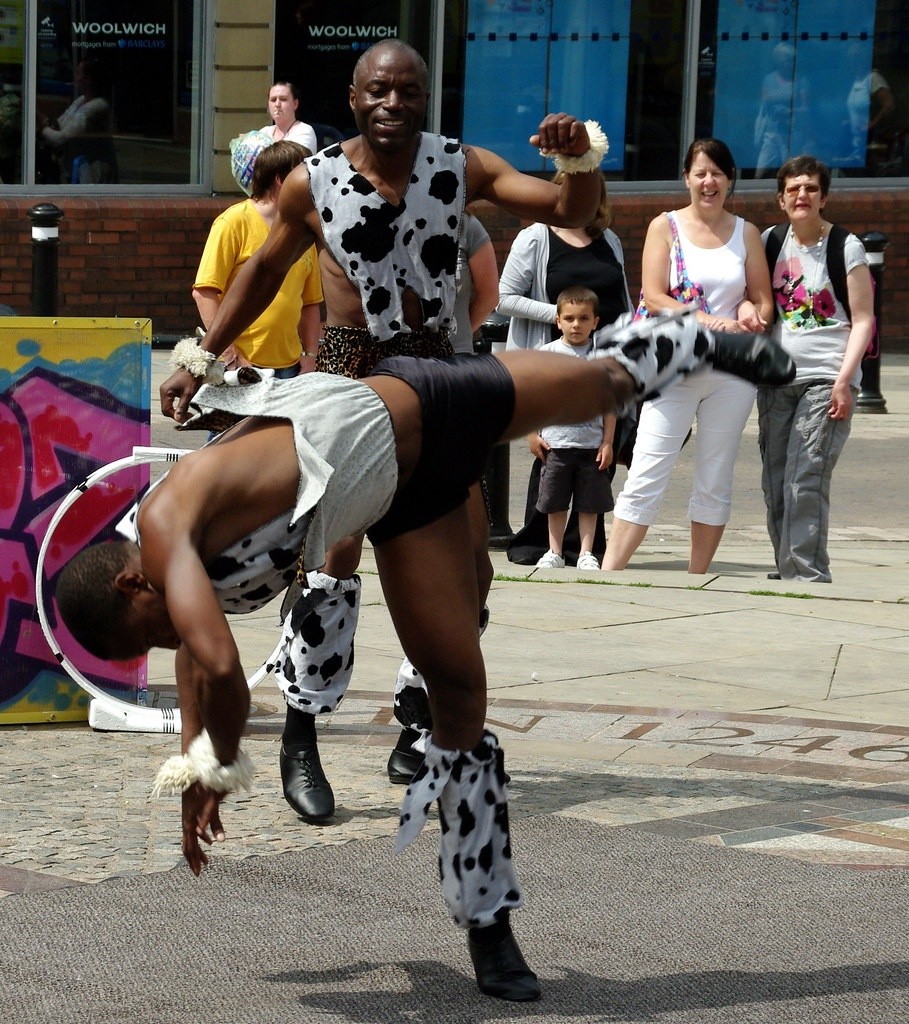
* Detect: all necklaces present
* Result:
[789,223,824,329]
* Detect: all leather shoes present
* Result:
[279,743,335,818]
[388,751,431,784]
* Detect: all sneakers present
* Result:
[535,549,566,569]
[577,551,600,570]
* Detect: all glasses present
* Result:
[784,184,826,198]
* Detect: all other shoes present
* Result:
[467,916,544,1002]
[697,328,797,386]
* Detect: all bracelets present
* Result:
[171,338,225,386]
[538,117,608,175]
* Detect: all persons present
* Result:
[736,153,874,583]
[258,80,318,156]
[832,44,895,178]
[525,288,615,569]
[603,137,774,577]
[54,314,799,1002]
[189,139,325,380]
[753,43,815,180]
[446,211,500,355]
[493,169,637,569]
[42,56,123,184]
[160,34,610,819]
